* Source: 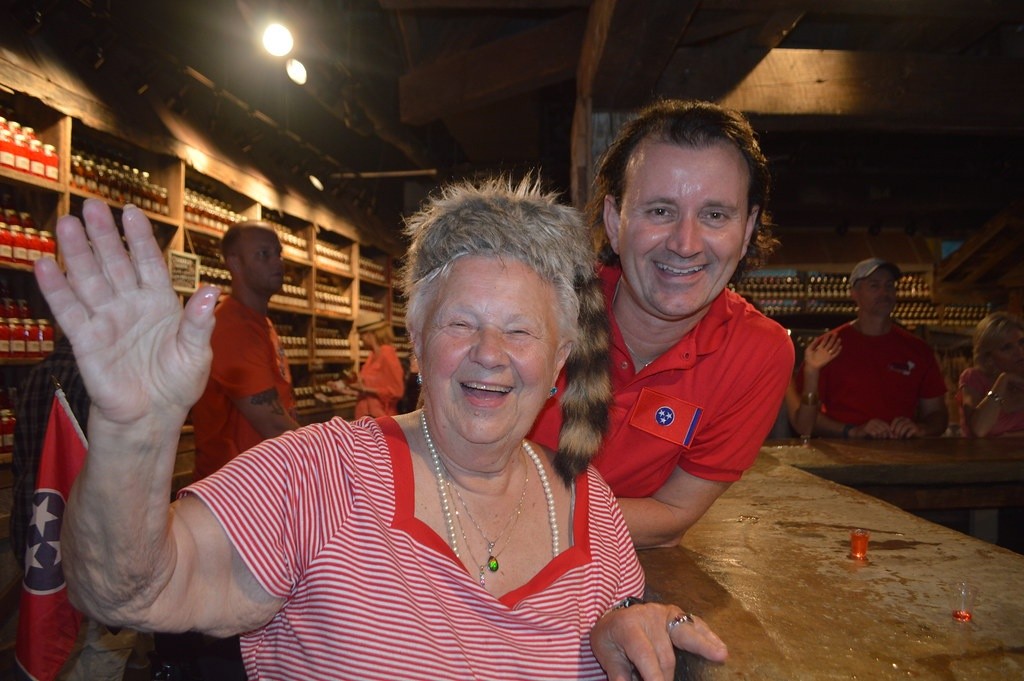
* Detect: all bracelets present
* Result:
[801,391,819,405]
[986,390,1008,406]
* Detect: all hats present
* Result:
[406,173,610,490]
[848,258,900,285]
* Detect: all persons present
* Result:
[742,294,842,438]
[10,334,138,681]
[33,175,729,681]
[330,313,420,420]
[195,220,300,483]
[796,258,949,439]
[527,102,793,549]
[958,313,1024,437]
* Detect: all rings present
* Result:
[828,349,834,354]
[667,613,695,633]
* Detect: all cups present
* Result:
[797,391,817,442]
[849,528,870,559]
[950,582,981,622]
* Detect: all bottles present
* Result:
[738,276,990,328]
[0,116,411,455]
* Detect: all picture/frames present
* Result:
[167,249,201,294]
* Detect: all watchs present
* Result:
[601,596,645,618]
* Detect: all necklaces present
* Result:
[613,278,648,366]
[419,406,559,589]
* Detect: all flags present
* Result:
[15,394,87,681]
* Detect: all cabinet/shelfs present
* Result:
[0,56,411,543]
